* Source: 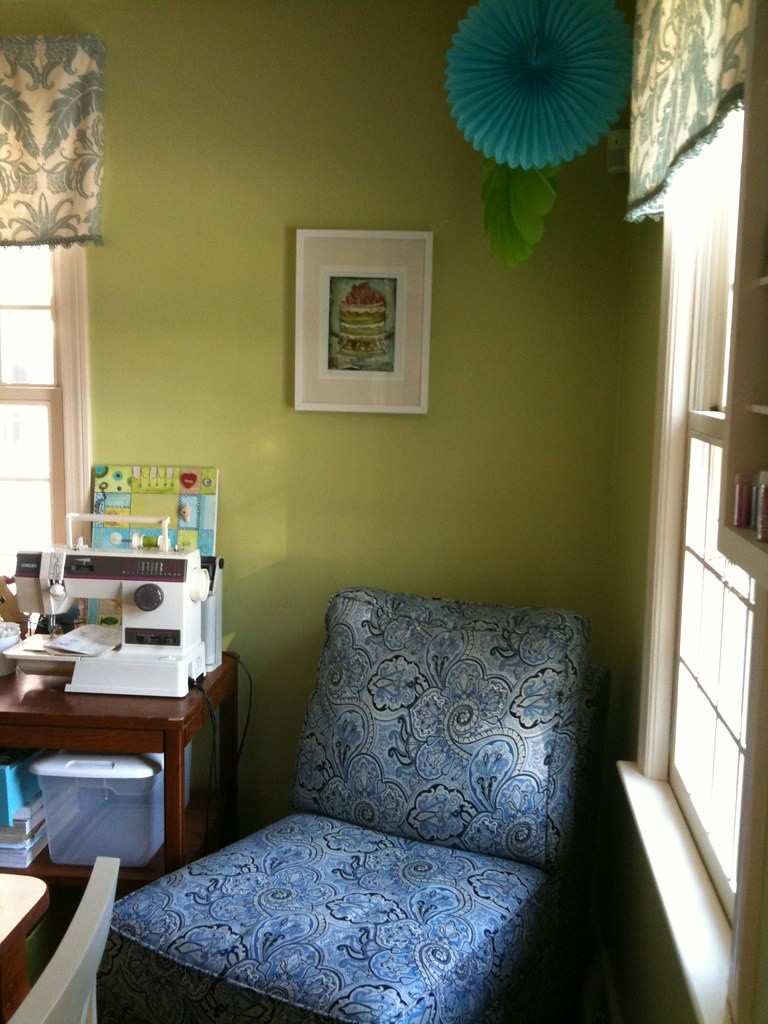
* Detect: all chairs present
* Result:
[94,585,615,1024]
[5,856,120,1024]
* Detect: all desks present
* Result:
[1,872,52,1024]
[1,649,238,883]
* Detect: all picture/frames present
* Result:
[293,229,434,415]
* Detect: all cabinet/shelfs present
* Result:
[715,0,768,585]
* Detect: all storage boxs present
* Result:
[28,739,191,870]
